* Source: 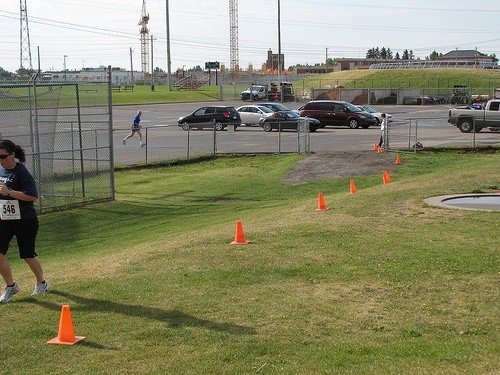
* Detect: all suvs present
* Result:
[298,100,377,128]
[177,106,242,132]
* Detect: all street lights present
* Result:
[64,54,68,81]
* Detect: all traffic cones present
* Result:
[47,305,87,345]
[348,180,358,195]
[382,169,392,184]
[373,143,384,153]
[395,156,401,165]
[230,220,251,245]
[314,193,330,211]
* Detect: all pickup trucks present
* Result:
[239,83,270,101]
[447,100,500,133]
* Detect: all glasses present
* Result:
[0,151,13,159]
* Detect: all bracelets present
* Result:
[8,189,11,196]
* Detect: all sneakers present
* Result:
[31,279,48,297]
[0,282,20,302]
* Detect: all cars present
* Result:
[253,103,300,118]
[259,111,321,132]
[354,105,393,126]
[233,105,277,126]
[468,102,485,110]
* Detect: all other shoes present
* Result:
[140,143,146,147]
[123,139,125,145]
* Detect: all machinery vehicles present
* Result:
[265,82,295,103]
[450,84,472,109]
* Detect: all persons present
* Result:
[0,139,49,303]
[123,110,146,148]
[378,113,387,147]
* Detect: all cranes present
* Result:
[229,0,240,85]
[137,0,151,86]
